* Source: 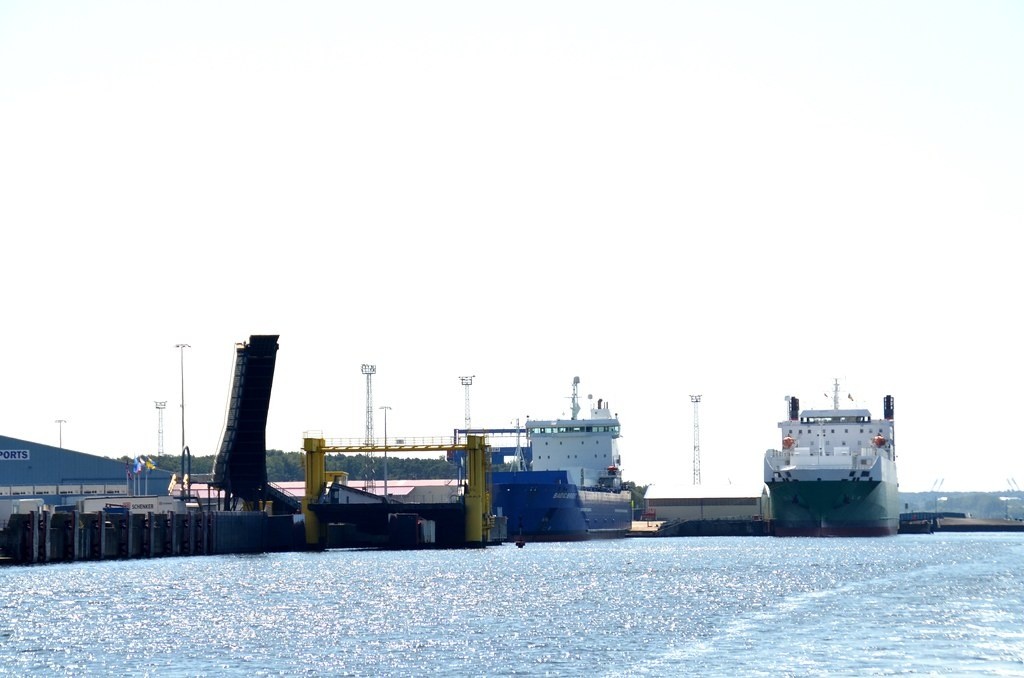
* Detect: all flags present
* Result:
[125,460,134,481]
[133,456,160,475]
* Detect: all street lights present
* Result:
[378,405,392,497]
[55,419,66,485]
[28,465,35,496]
[174,342,192,448]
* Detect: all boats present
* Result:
[753,376,903,537]
